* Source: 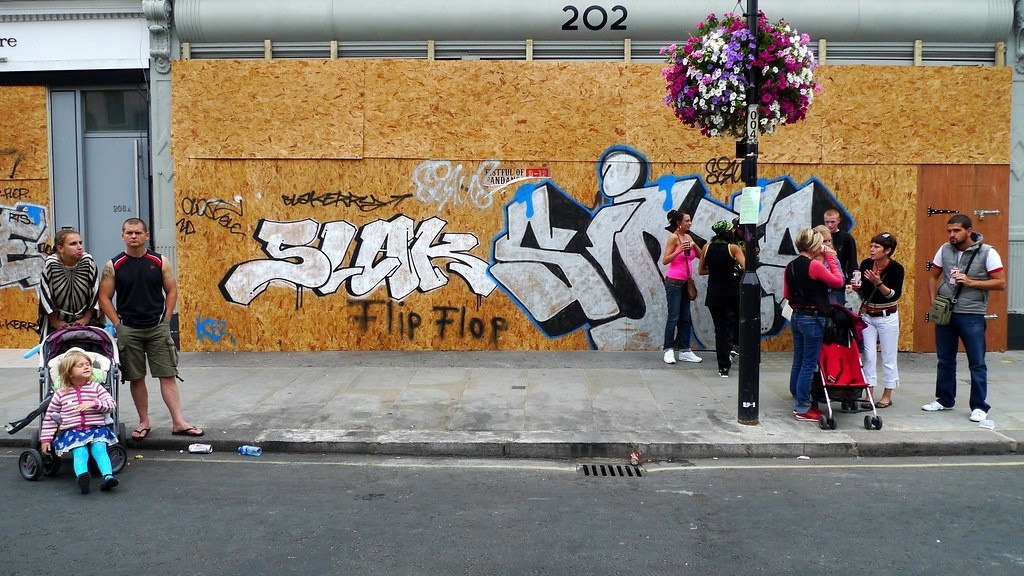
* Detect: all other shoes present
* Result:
[861,398,874,409]
[874,400,893,408]
[730,345,741,355]
[718,368,729,378]
[77,471,91,495]
[99,474,120,490]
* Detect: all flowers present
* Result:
[659,7,820,140]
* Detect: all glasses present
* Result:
[882,233,891,239]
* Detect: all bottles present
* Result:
[104,322,115,337]
[238,445,262,457]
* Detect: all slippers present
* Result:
[131,428,151,439]
[172,425,204,437]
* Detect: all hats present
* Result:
[709,219,734,242]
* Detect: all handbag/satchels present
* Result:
[928,294,953,325]
[686,278,698,301]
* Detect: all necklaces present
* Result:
[875,262,883,272]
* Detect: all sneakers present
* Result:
[678,351,703,363]
[968,408,987,421]
[663,350,677,364]
[793,408,824,422]
[921,401,955,411]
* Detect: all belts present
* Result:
[865,310,897,317]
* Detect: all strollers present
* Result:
[812,305,883,430]
[19,313,128,482]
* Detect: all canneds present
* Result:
[684,241,691,256]
[188,444,213,454]
[949,268,960,285]
[853,269,861,288]
[630,453,638,465]
[819,245,825,253]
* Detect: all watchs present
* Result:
[874,281,883,288]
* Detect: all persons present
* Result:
[921,214,1006,422]
[98,218,205,440]
[663,210,703,364]
[40,350,120,494]
[784,209,858,422]
[698,215,761,378]
[851,232,904,409]
[37,230,108,343]
